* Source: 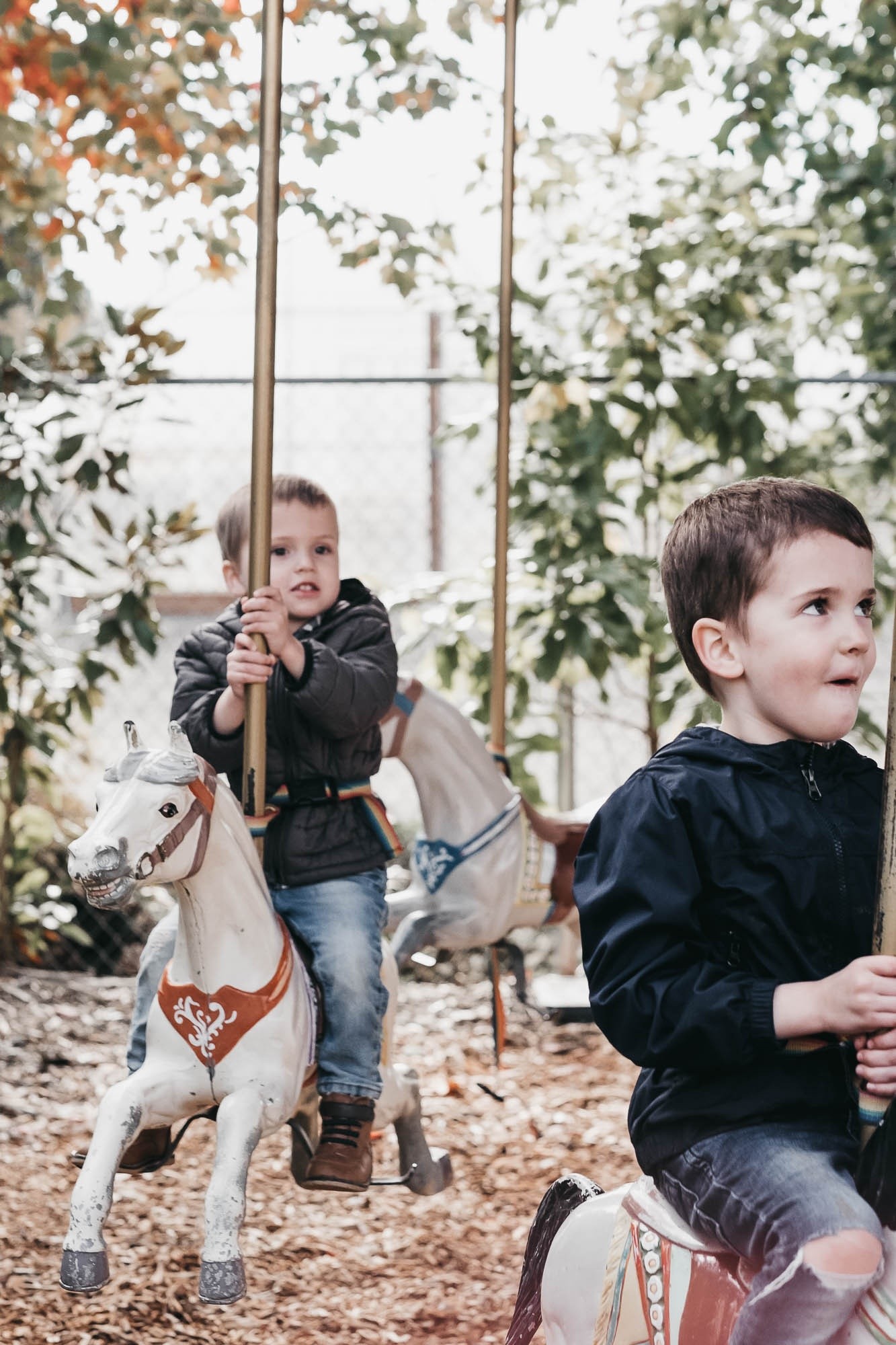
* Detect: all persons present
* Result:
[573,475,895,1345]
[59,475,399,1195]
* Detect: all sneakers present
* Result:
[70,1119,176,1174]
[304,1091,372,1191]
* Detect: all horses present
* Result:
[58,720,454,1305]
[504,1095,896,1345]
[376,674,613,985]
